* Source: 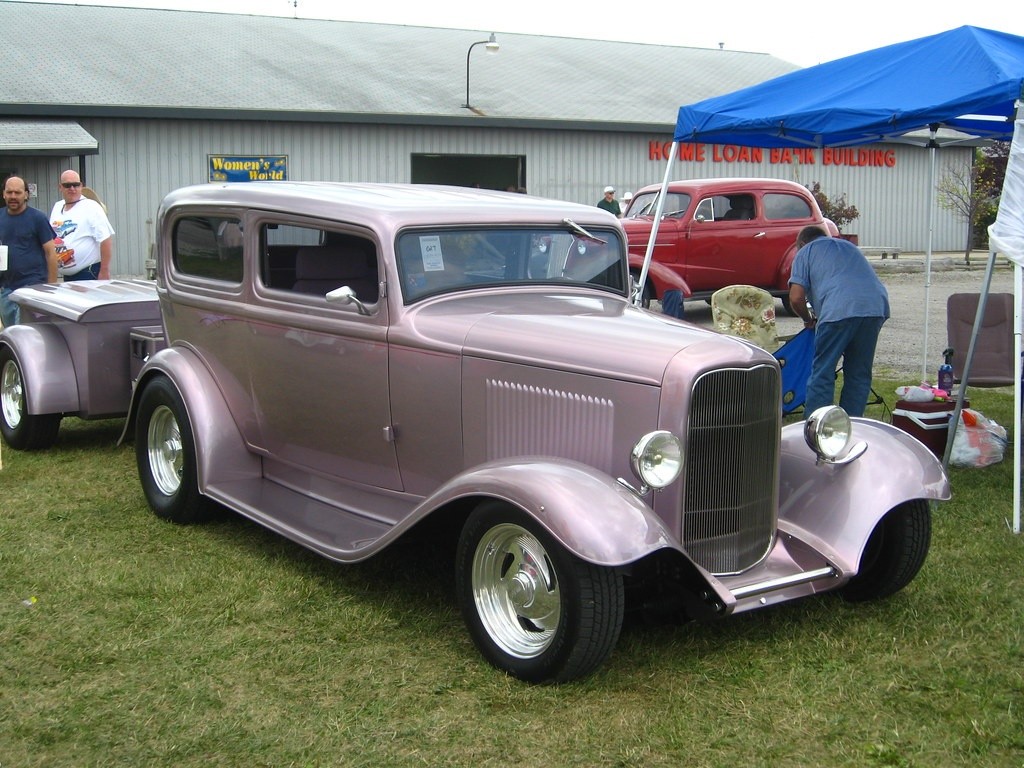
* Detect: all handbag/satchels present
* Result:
[947,407,1008,469]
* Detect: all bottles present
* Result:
[938,348,953,396]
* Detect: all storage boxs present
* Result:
[891,400,970,451]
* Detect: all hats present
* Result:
[604,186,616,193]
[620,192,633,200]
[81,186,108,215]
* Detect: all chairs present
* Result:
[943,293,1014,388]
[710,284,802,369]
[723,194,755,220]
[291,245,379,304]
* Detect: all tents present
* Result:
[635,26,1024,534]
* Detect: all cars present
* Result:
[1,180,955,680]
[527,177,859,317]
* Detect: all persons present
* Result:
[597,186,633,217]
[788,226,889,419]
[0,177,57,328]
[49,170,115,282]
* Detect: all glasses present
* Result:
[61,182,81,188]
[609,192,615,195]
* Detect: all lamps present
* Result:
[460,32,499,108]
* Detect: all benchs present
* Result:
[225,243,325,290]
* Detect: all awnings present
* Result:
[0,118,101,188]
